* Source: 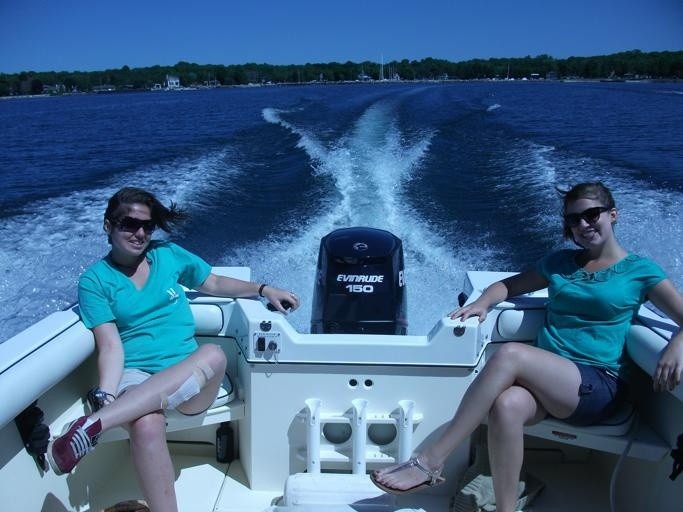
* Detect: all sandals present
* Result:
[370,456,446,495]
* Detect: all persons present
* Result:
[369,183,683,511]
[46,186,299,511]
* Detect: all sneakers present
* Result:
[47,415,103,476]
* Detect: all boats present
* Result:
[0,261,683,512]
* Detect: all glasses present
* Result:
[564,207,607,228]
[110,218,155,234]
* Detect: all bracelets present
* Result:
[105,393,116,400]
[259,284,266,297]
